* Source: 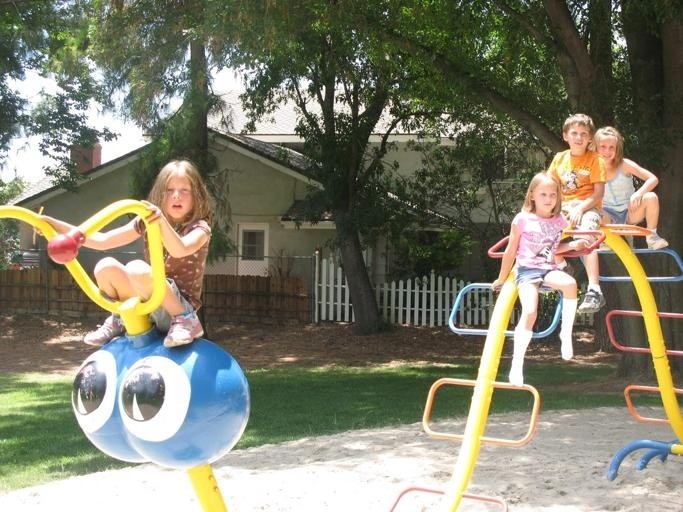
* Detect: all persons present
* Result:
[29,160,215,347]
[544,113,607,315]
[588,125,670,249]
[491,170,589,387]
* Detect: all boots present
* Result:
[508,327,533,388]
[559,298,577,361]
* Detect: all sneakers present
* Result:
[647,234,669,249]
[578,289,606,313]
[84,316,126,346]
[165,311,204,348]
